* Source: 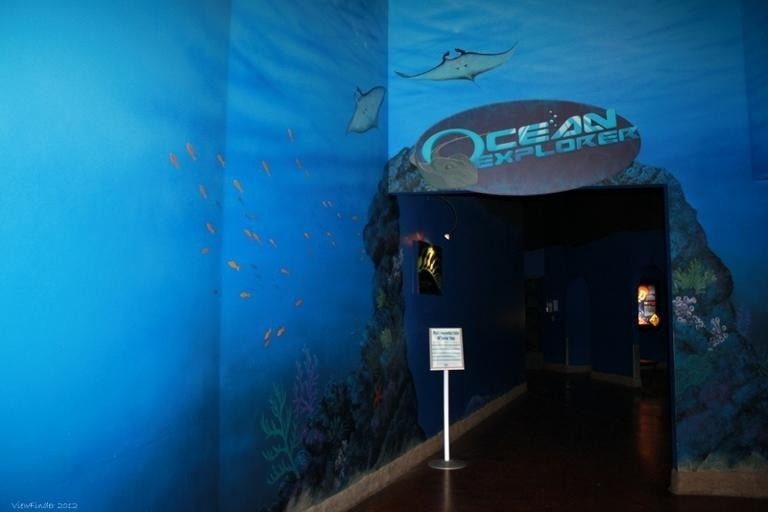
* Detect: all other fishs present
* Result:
[168,127,357,349]
